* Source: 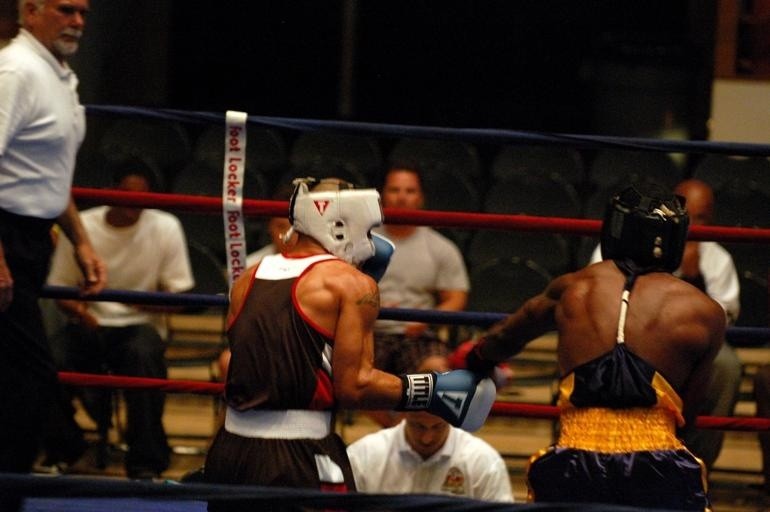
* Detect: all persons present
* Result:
[219,187,298,391]
[346,411,516,504]
[202,176,497,512]
[449,181,726,511]
[0,0,107,511]
[37,157,196,480]
[363,169,470,427]
[551,180,742,470]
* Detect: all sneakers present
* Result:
[69,447,99,475]
[121,456,161,479]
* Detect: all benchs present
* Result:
[708,349,766,490]
[73,313,230,481]
[337,331,558,488]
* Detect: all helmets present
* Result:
[286,174,383,268]
[599,178,692,275]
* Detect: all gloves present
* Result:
[358,231,396,285]
[443,330,509,393]
[393,367,499,433]
